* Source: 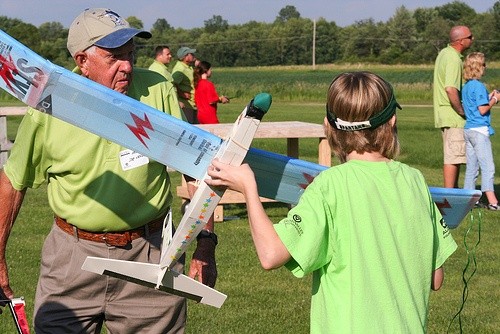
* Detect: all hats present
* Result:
[66,8,152,57]
[177,47,196,60]
[326,85,403,131]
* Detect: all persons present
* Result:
[192,59,230,124]
[462,52,500,211]
[203,71,459,334]
[433,26,475,188]
[0,4,218,334]
[172,45,201,124]
[149,45,186,110]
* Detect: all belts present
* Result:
[53,207,170,248]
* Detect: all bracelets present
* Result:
[492,96,498,104]
[196,229,218,245]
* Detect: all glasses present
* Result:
[458,34,472,42]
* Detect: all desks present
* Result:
[176,122,333,222]
[0,107,34,169]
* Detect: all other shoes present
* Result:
[487,201,500,211]
[473,201,483,207]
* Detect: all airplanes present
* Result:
[0,29,482,308]
